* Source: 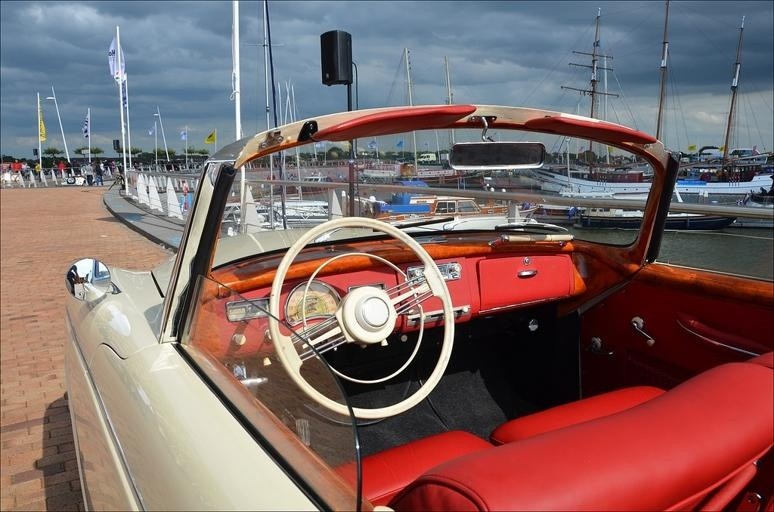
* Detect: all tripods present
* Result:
[108,150,125,191]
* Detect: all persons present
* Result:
[0,157,140,187]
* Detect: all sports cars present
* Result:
[62,103,774,507]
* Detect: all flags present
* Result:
[204,130,216,144]
[368,139,375,151]
[180,129,187,141]
[82,115,88,138]
[396,140,403,148]
[148,125,156,135]
[688,144,697,152]
[578,145,584,153]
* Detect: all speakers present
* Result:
[321,30,353,86]
[113,139,119,150]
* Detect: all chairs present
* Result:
[331,351,774,512]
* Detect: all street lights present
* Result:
[154,113,159,164]
[37,91,56,169]
